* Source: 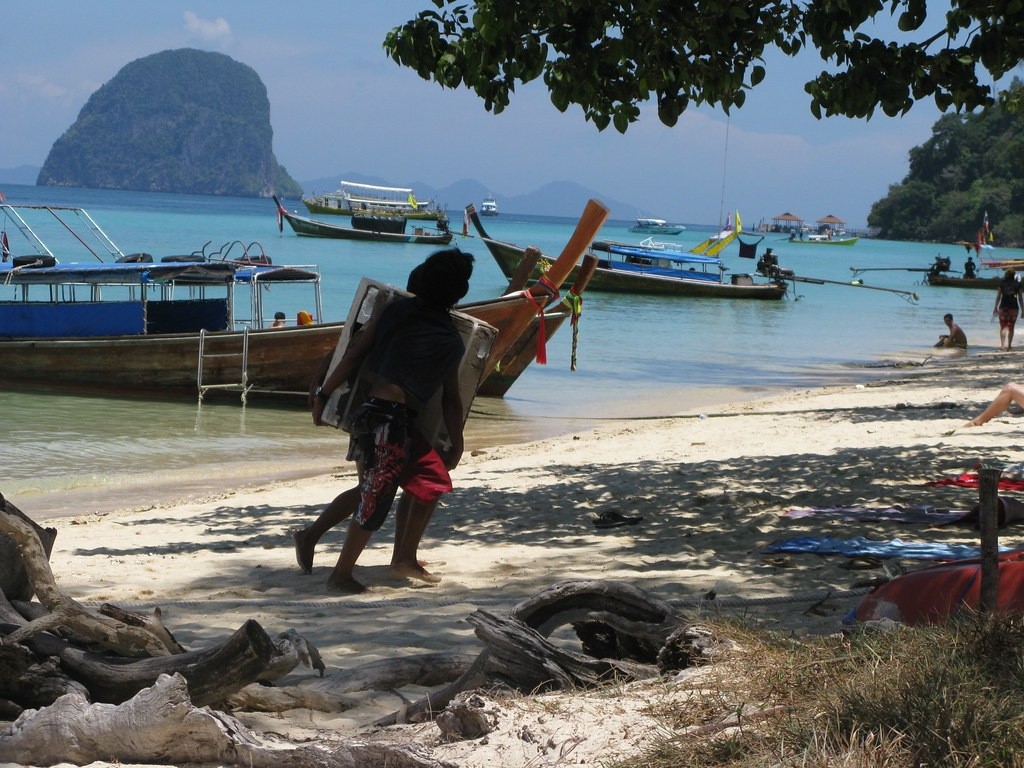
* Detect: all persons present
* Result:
[800,231,803,241]
[297,312,311,325]
[271,312,286,327]
[963,257,976,278]
[294,249,473,593]
[993,268,1024,350]
[935,314,967,349]
[963,382,1024,427]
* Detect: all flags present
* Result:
[725,210,742,233]
[408,196,417,207]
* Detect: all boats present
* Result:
[0,194,612,409]
[926,258,1024,295]
[1,204,605,396]
[270,179,456,245]
[627,209,688,235]
[478,193,501,216]
[787,227,860,246]
[465,201,796,301]
[838,551,1023,635]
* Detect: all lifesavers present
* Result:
[114,252,153,264]
[234,255,272,267]
[12,255,56,269]
[161,255,204,263]
